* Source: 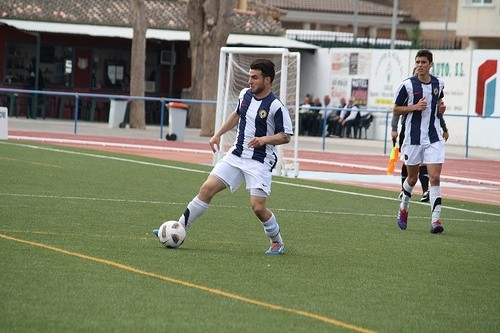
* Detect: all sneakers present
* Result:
[430,220,444,234]
[265,240,285,255]
[397,204,409,230]
[152,230,158,236]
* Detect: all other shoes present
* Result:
[420,190,429,202]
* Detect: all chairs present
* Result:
[350,113,373,139]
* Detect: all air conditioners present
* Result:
[160,51,177,66]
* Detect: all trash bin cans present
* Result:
[109,98,131,128]
[165,102,188,142]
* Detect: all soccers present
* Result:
[158,220,186,248]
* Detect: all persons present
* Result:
[392,49,449,233]
[299,94,361,138]
[152,58,294,255]
